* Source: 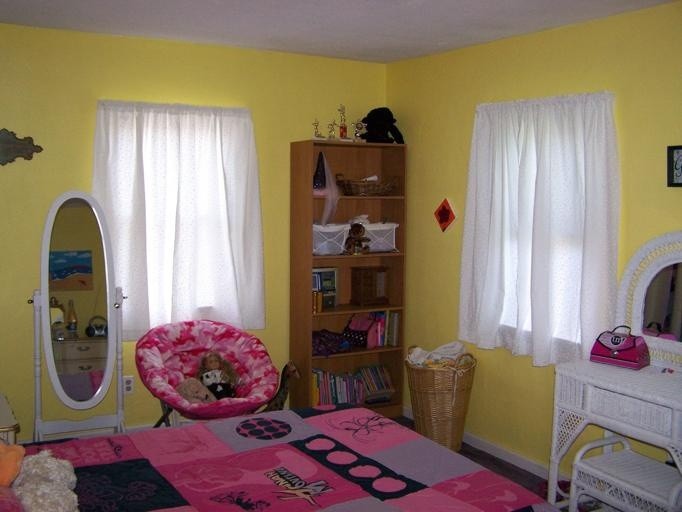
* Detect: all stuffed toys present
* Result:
[12,449,80,511]
[343,223,372,255]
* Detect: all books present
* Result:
[311,266,390,311]
[340,310,400,350]
[312,362,395,405]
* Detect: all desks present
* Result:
[547,356,682,509]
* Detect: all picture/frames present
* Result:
[666,145,682,187]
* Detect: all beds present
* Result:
[10,406,558,511]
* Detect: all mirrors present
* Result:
[27,189,129,441]
[613,230,682,373]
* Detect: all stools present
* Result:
[567,437,682,512]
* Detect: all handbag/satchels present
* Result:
[589,327,649,370]
[344,313,377,350]
[643,322,677,341]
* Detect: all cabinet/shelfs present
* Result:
[51,340,107,374]
[288,138,408,415]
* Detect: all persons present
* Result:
[197,352,247,401]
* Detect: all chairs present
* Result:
[136,319,280,429]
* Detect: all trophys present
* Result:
[328,120,338,139]
[312,117,327,139]
[353,119,368,143]
[337,104,353,143]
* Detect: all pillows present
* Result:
[0,436,81,512]
[59,372,104,400]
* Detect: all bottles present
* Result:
[66,299,78,340]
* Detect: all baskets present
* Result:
[404,344,478,451]
[335,171,400,196]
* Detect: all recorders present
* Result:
[86,324,108,337]
